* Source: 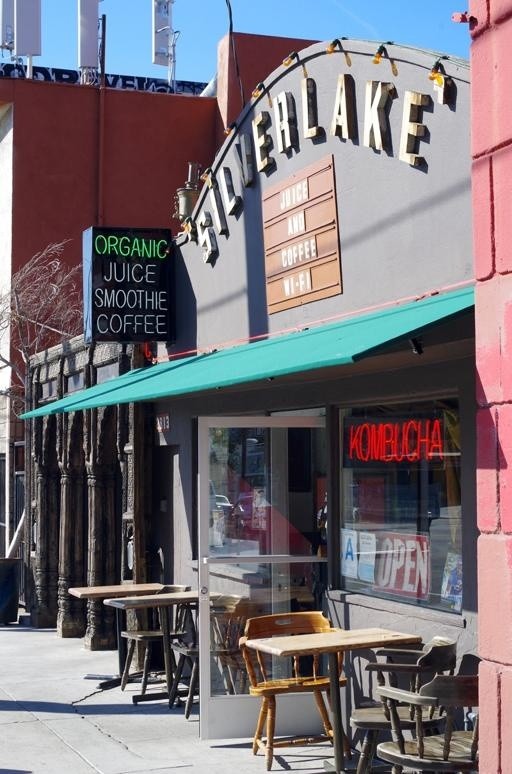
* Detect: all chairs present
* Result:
[375,653,483,774]
[119,583,221,695]
[346,635,458,773]
[168,593,250,719]
[238,609,355,772]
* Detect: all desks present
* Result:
[102,590,224,706]
[245,626,424,774]
[67,582,167,689]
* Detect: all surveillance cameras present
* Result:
[410,334,427,357]
